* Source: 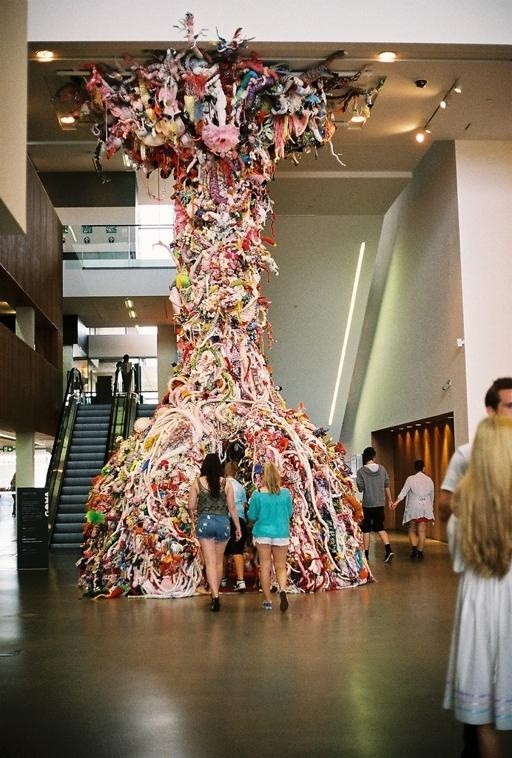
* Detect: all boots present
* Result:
[410,546,423,559]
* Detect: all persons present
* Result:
[118,354,132,391]
[186,453,242,612]
[221,461,250,591]
[439,413,512,758]
[436,376,512,578]
[391,460,436,559]
[355,446,396,563]
[243,461,295,612]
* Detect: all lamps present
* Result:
[414,75,465,144]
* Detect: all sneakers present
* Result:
[210,600,221,612]
[384,551,394,563]
[280,590,288,611]
[221,579,227,587]
[263,601,272,610]
[233,580,246,591]
